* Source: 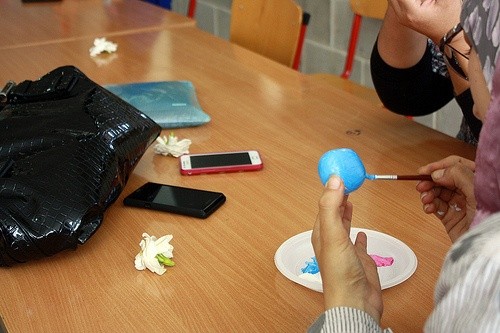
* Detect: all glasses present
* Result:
[444,23,472,62]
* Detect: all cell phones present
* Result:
[123,182,226,218]
[180,150,263,176]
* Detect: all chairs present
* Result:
[340,0,416,120]
[229,0,312,69]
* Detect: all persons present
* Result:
[307,0,500,333]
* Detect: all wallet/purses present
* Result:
[105,80,212,127]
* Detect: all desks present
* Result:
[0,24,477,333]
[0,0,196,50]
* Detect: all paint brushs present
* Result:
[366,173,433,180]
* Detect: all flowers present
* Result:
[89,38,118,57]
[134,233,176,276]
[154,131,192,159]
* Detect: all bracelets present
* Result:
[438,22,464,54]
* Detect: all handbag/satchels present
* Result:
[0,64,163,267]
